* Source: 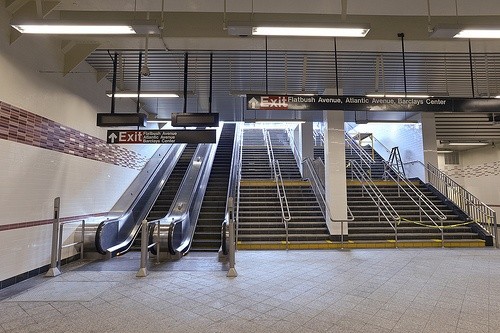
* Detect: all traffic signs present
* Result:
[107,129,218,146]
[244,94,496,112]
